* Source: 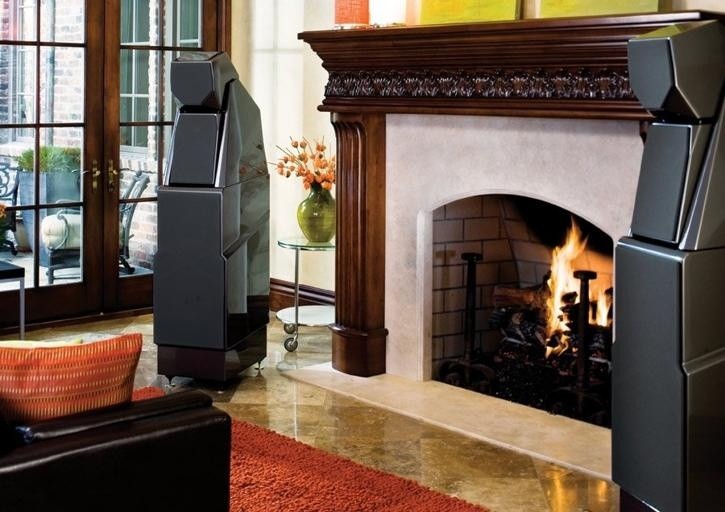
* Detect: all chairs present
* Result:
[0,260,232,512]
[0,151,151,286]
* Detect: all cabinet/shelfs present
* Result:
[270,227,348,352]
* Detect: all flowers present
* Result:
[265,133,343,192]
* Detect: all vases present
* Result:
[290,188,345,244]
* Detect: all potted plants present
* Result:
[15,147,82,267]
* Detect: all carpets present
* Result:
[129,370,495,512]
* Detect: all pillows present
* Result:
[0,332,144,420]
